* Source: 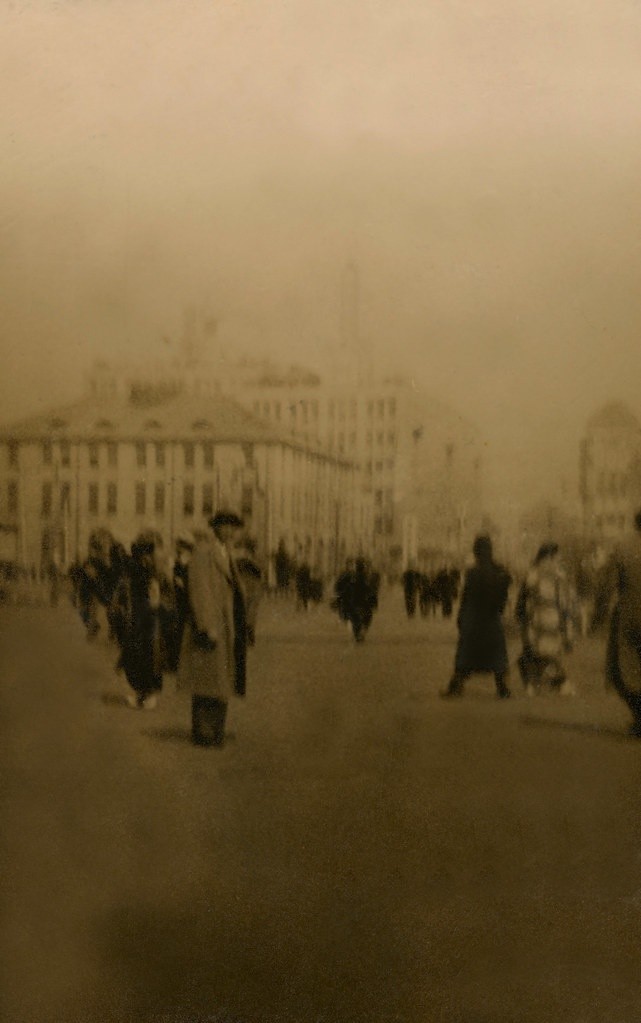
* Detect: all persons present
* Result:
[184,506,255,748]
[68,526,192,706]
[330,552,383,638]
[268,537,326,610]
[437,530,513,701]
[513,540,587,697]
[402,552,462,621]
[587,508,641,741]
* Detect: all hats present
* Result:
[209,509,244,529]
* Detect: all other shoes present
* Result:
[525,681,541,697]
[559,679,573,695]
